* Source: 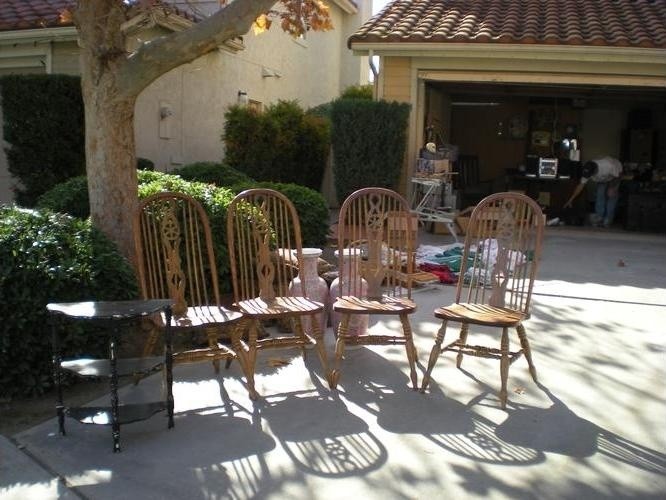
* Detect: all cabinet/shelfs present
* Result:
[504,173,588,226]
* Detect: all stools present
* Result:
[45,298,173,453]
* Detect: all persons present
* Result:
[563,157,624,232]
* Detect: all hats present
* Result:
[582,161,598,178]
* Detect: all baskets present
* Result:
[457,207,486,237]
